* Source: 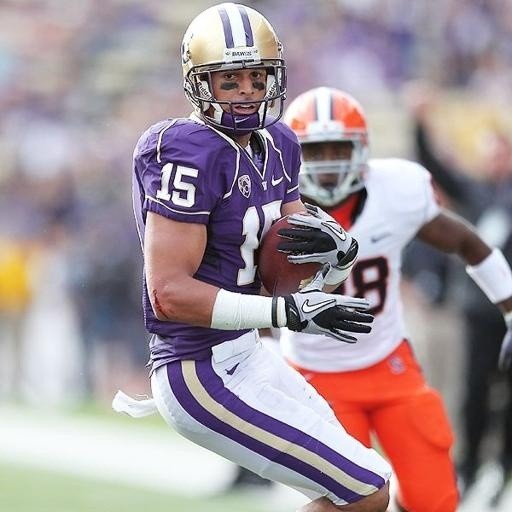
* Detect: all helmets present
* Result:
[284,88,370,207]
[181,4,287,135]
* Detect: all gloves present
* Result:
[271,261,374,343]
[277,202,359,285]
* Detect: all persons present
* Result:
[258,86,511,511]
[130,2,390,510]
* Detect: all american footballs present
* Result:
[256,212,323,297]
